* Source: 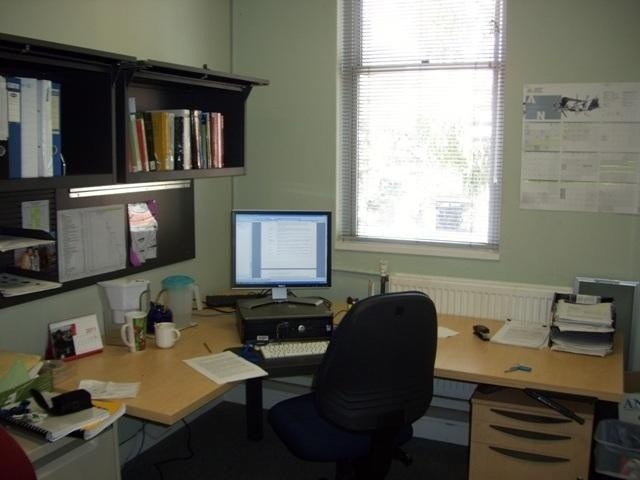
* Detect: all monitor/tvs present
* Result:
[231,210,332,309]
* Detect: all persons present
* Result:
[21,245,49,272]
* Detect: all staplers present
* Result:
[473,325,490,342]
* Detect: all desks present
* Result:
[44,293,624,441]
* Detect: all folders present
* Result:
[51,83,62,177]
[4,77,21,179]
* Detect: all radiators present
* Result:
[389,273,573,402]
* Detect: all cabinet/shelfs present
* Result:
[9,423,123,479]
[1,31,136,191]
[466,384,595,480]
[121,58,271,182]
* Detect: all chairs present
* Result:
[268,292,438,480]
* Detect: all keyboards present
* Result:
[261,340,331,361]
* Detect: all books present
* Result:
[0,390,126,443]
[128,97,225,173]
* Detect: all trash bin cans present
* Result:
[593,419,640,480]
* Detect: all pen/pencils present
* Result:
[203,342,212,353]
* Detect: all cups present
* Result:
[162,275,202,331]
[155,322,180,349]
[120,311,148,353]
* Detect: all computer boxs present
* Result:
[235,296,336,344]
